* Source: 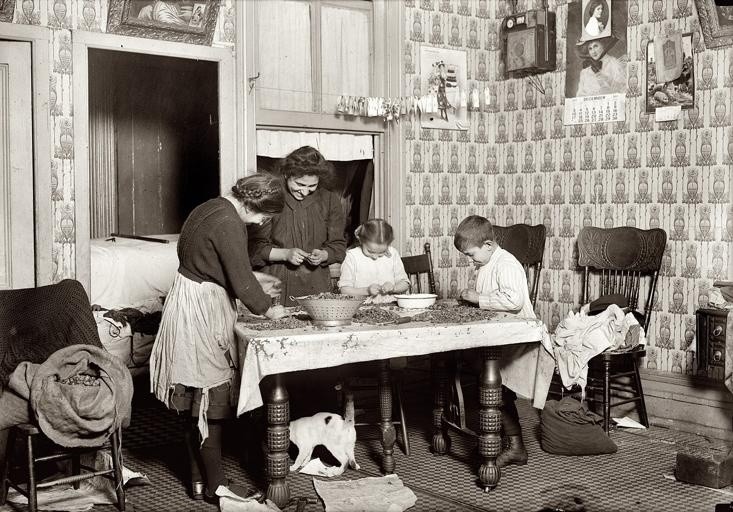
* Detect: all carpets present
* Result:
[85,374,733,512]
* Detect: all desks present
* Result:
[221,295,546,509]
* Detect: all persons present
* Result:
[453,215,540,466]
[248,146,348,424]
[585,1,605,35]
[336,220,412,382]
[575,34,627,99]
[149,171,288,506]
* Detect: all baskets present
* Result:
[289,293,375,326]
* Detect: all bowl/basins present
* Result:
[393,293,437,309]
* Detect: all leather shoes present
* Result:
[203,479,263,505]
[192,478,234,499]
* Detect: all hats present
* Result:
[588,294,629,315]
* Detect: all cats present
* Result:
[287,411,361,475]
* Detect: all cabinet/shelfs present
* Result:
[692,304,733,393]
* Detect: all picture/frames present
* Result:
[419,44,469,131]
[105,1,223,47]
[692,1,733,50]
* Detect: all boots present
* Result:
[496,435,528,468]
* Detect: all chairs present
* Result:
[488,220,546,319]
[399,243,440,296]
[0,277,126,512]
[534,224,668,441]
